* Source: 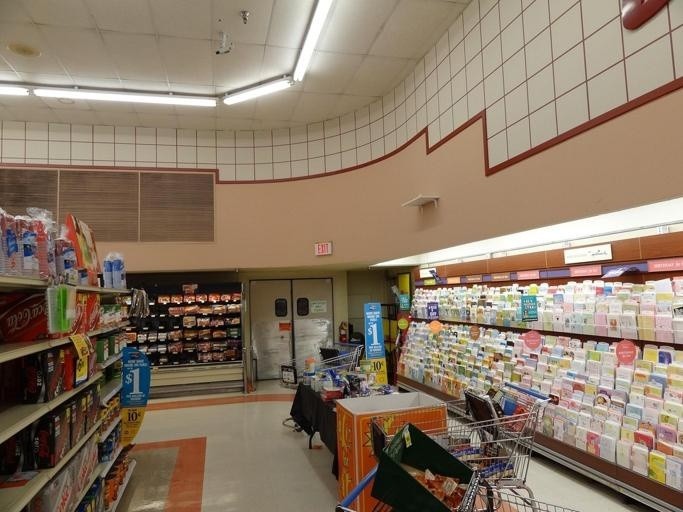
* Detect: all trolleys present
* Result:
[334,452,581,512]
[368,381,559,511]
[275,341,365,434]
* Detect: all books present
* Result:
[410,277,683,345]
[397,321,683,491]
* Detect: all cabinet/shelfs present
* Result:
[1,272,141,512]
[393,283,683,512]
